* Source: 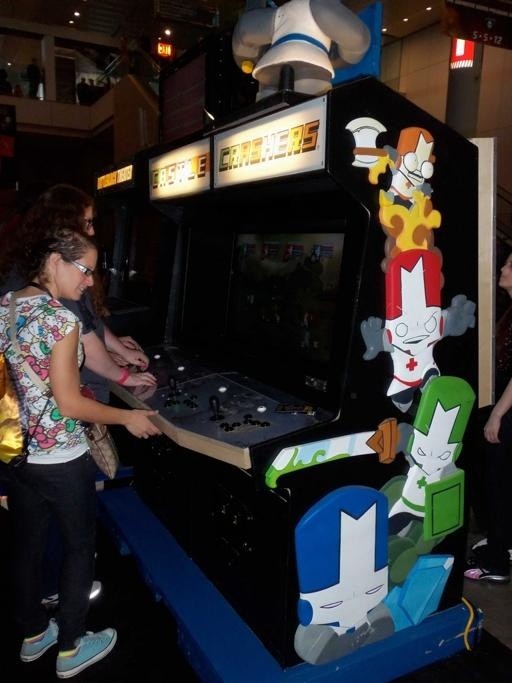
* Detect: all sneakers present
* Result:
[42,581,102,608]
[464,568,509,583]
[19,620,59,662]
[56,628,117,678]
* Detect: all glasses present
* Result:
[73,262,95,277]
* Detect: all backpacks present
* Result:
[1,301,63,470]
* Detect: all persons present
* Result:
[0,184,163,683]
[0,64,25,98]
[104,77,115,87]
[23,57,42,100]
[88,78,95,86]
[463,250,512,586]
[76,76,89,106]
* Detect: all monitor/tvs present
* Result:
[226,231,346,362]
[109,214,178,343]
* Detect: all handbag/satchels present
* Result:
[84,421,120,479]
